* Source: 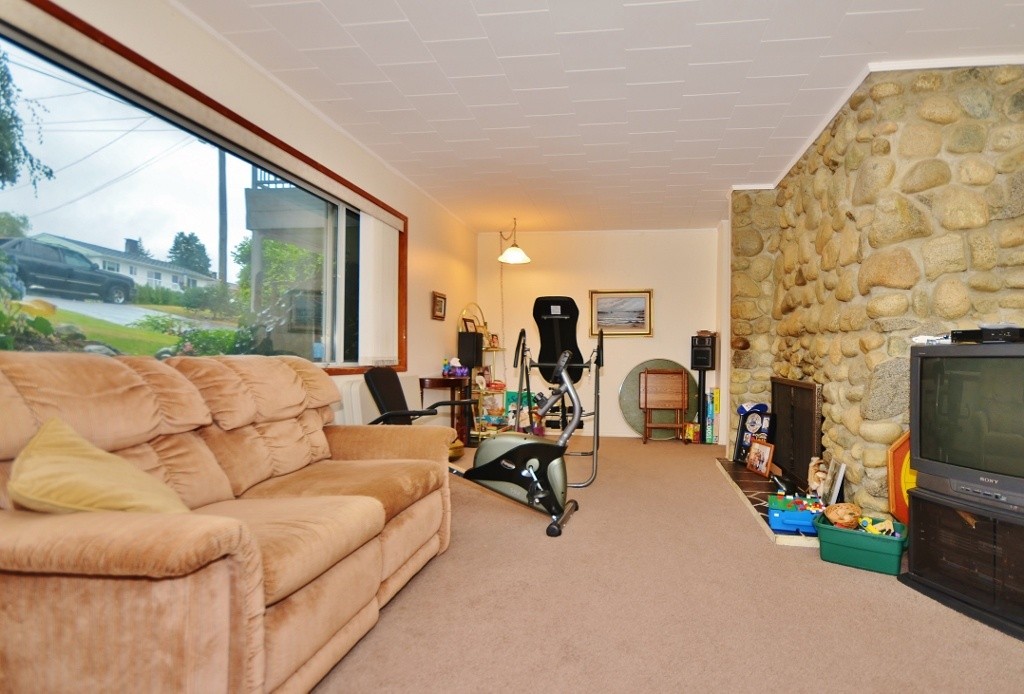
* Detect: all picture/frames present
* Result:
[733,411,774,467]
[821,459,848,508]
[587,287,655,338]
[464,318,477,334]
[431,290,448,322]
[747,439,775,478]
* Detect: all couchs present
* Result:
[0,349,459,694]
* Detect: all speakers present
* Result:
[458,330,483,367]
[690,336,715,370]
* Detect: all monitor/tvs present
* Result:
[911,341,1024,515]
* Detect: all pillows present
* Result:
[6,419,192,518]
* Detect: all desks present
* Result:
[419,377,471,447]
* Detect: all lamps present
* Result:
[499,219,533,265]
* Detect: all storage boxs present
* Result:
[768,509,822,536]
[684,388,720,445]
[815,511,909,576]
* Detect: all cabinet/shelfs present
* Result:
[898,491,1024,643]
[459,302,508,442]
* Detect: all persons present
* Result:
[491,335,499,348]
[749,448,765,472]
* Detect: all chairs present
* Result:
[364,365,478,427]
